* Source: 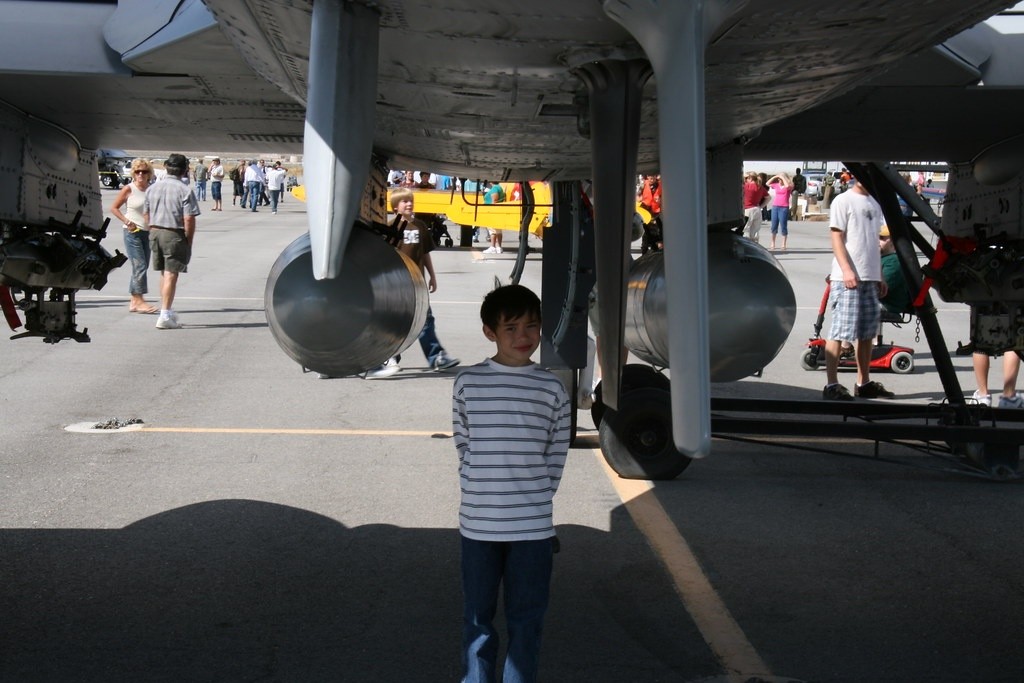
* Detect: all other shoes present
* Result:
[211,208,222,211]
[263,202,270,206]
[474,239,480,242]
[232,199,235,206]
[257,204,261,206]
[129,303,159,313]
[239,199,242,206]
[252,210,258,212]
[272,211,277,214]
[483,247,503,253]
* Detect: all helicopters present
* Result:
[294,175,654,247]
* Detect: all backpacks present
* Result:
[229,164,242,181]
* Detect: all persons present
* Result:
[385,163,952,256]
[111,164,119,189]
[232,155,289,214]
[110,158,160,314]
[143,154,201,329]
[822,175,896,402]
[904,172,932,199]
[451,285,572,683]
[838,224,902,359]
[970,307,1024,410]
[317,187,460,379]
[192,158,209,201]
[207,158,225,211]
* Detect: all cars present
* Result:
[802,173,832,196]
[830,170,854,195]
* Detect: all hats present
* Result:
[212,158,220,161]
[163,153,189,170]
[879,223,890,236]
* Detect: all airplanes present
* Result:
[1,0,1024,491]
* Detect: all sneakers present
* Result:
[155,312,183,328]
[997,395,1024,409]
[822,385,856,401]
[854,380,894,398]
[432,350,462,371]
[969,390,992,407]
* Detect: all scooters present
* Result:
[800,273,916,374]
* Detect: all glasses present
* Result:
[134,169,150,174]
